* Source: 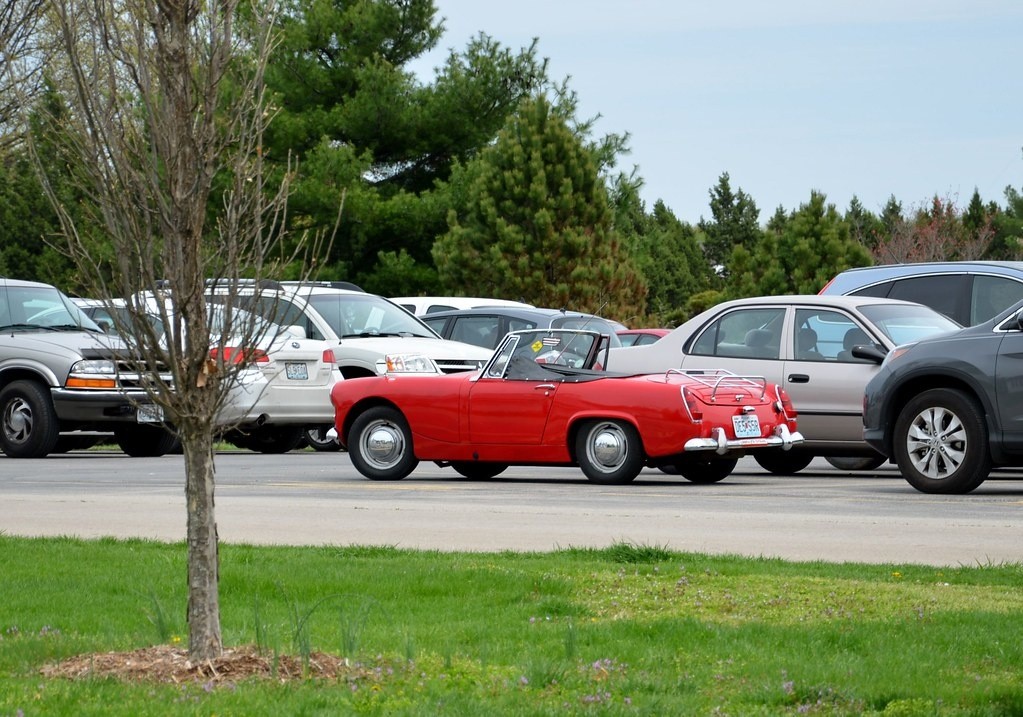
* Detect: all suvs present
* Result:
[0,277,496,459]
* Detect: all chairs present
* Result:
[835,328,869,362]
[797,329,825,360]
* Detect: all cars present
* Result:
[331,262,1022,484]
[862,296,1023,495]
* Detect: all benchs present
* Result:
[695,328,780,358]
